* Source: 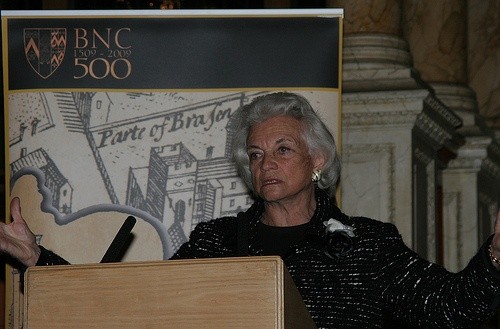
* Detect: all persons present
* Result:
[0,92,500,329]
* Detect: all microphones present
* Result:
[100,215,136,264]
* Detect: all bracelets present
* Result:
[488,244,500,266]
